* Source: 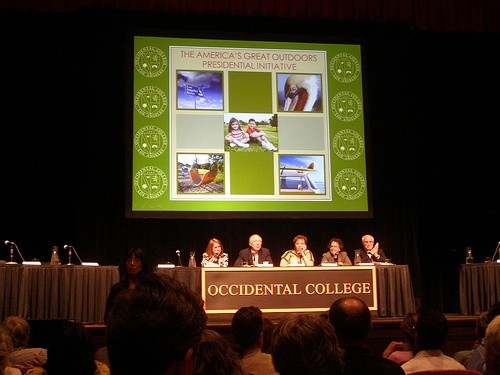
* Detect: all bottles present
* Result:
[188,251,196,267]
[354,250,361,266]
[51,246,59,264]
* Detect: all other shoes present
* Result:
[262,143,277,151]
[241,144,249,147]
[230,143,236,147]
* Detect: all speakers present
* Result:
[5,240,15,245]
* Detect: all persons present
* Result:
[233,234,272,266]
[382,312,466,375]
[329,297,406,375]
[231,306,279,375]
[271,314,344,375]
[202,238,228,268]
[454,301,500,375]
[0,316,110,375]
[225,118,277,151]
[106,272,240,375]
[280,235,315,266]
[104,248,148,324]
[321,238,352,265]
[355,235,386,263]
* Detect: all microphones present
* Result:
[64,245,73,249]
[176,250,180,256]
[251,250,255,261]
[333,252,339,255]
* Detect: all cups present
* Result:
[243,262,247,267]
[466,257,473,263]
[485,257,491,263]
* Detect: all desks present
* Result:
[459,262,500,314]
[0,263,417,324]
